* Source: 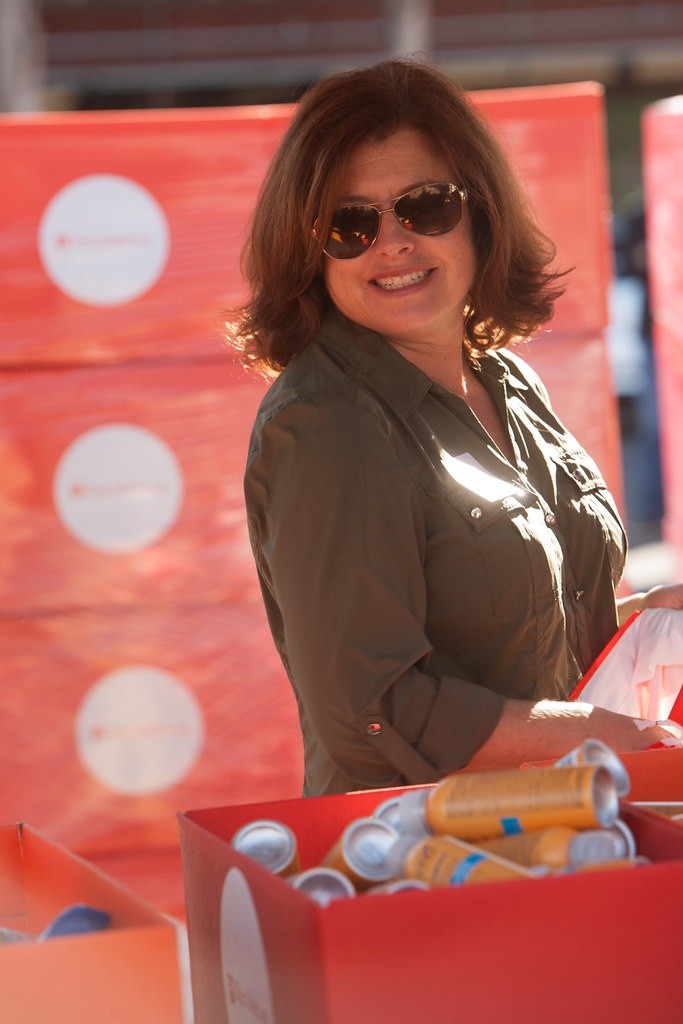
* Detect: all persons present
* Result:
[231,60,682,799]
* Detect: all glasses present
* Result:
[309,181,471,261]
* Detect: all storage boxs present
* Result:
[520,746,683,830]
[0,89,625,918]
[0,822,184,1024]
[176,783,683,1024]
[643,94,682,554]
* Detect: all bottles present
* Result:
[227,737,657,907]
